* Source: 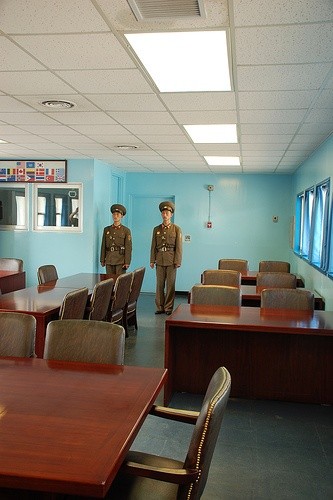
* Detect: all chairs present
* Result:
[0,258,315,500]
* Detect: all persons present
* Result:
[100,204,132,274]
[150,201,182,315]
[70,208,78,227]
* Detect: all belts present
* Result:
[106,247,120,251]
[158,247,175,252]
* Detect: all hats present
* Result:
[110,204,126,216]
[159,201,174,213]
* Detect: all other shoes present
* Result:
[166,311,171,315]
[155,311,164,314]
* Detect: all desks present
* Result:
[201,269,305,289]
[0,270,25,294]
[163,304,333,408]
[0,273,122,358]
[0,355,169,500]
[188,284,325,310]
